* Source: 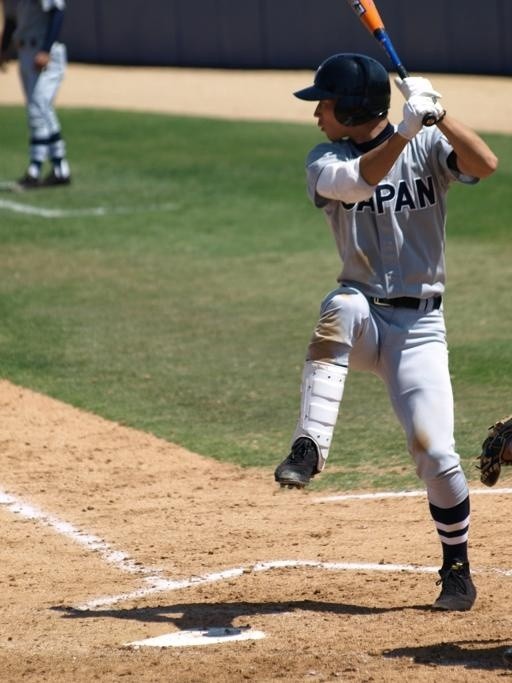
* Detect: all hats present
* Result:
[290,53,390,119]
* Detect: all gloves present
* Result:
[390,76,446,140]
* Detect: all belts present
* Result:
[339,282,441,310]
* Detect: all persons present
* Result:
[272,50,498,610]
[0,0,72,187]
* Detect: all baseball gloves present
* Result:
[475,415,512,486]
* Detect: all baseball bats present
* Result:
[347,0,437,126]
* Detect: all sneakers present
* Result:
[15,173,71,189]
[273,435,319,489]
[429,561,476,611]
